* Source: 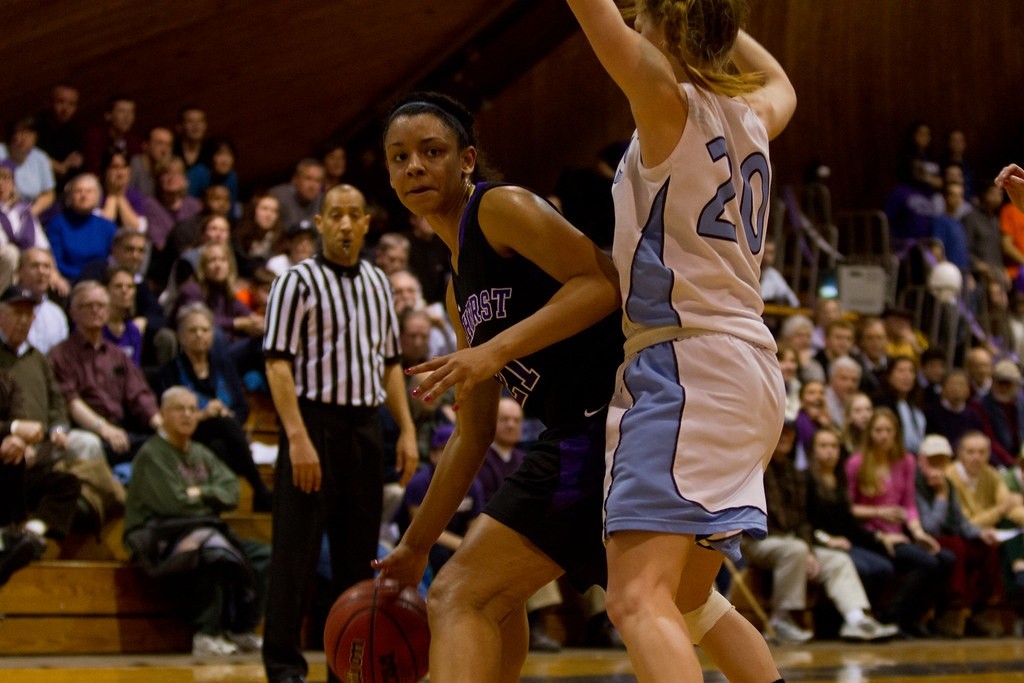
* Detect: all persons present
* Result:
[713,124,1024,644]
[565,0,798,683]
[369,100,629,682]
[0,81,627,660]
[263,186,418,683]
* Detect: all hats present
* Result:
[918,436,954,457]
[0,284,40,305]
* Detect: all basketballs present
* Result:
[321,577,430,683]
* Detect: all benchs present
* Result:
[0,390,281,656]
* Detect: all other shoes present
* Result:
[765,618,813,647]
[601,622,622,645]
[899,608,1004,638]
[528,627,559,649]
[226,631,262,652]
[842,617,899,640]
[194,633,240,665]
